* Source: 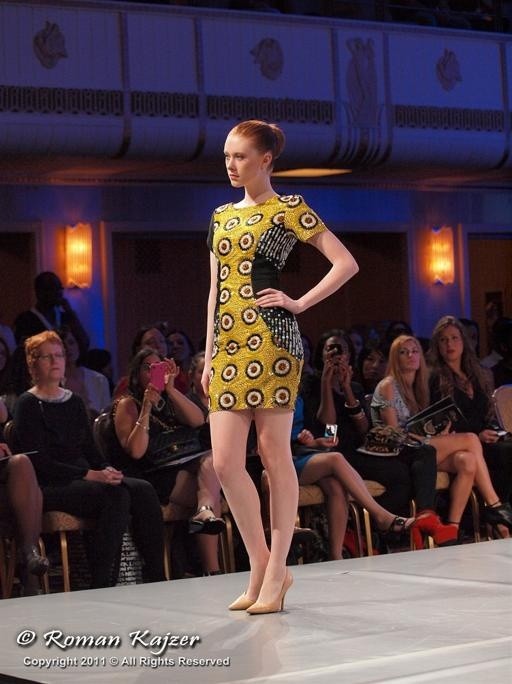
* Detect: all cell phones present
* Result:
[330,342,341,355]
[151,362,165,389]
[325,424,337,441]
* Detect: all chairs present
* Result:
[0,368,512,598]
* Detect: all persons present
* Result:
[1,271,511,595]
[198,119,360,613]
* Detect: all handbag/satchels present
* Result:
[143,424,201,466]
[366,423,424,455]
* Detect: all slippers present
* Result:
[378,515,415,536]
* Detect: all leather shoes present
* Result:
[293,527,310,542]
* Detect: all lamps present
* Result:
[428,224,455,285]
[65,222,92,288]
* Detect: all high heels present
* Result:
[18,544,48,583]
[228,591,256,610]
[479,504,511,538]
[248,566,292,613]
[189,504,225,536]
[412,510,458,551]
